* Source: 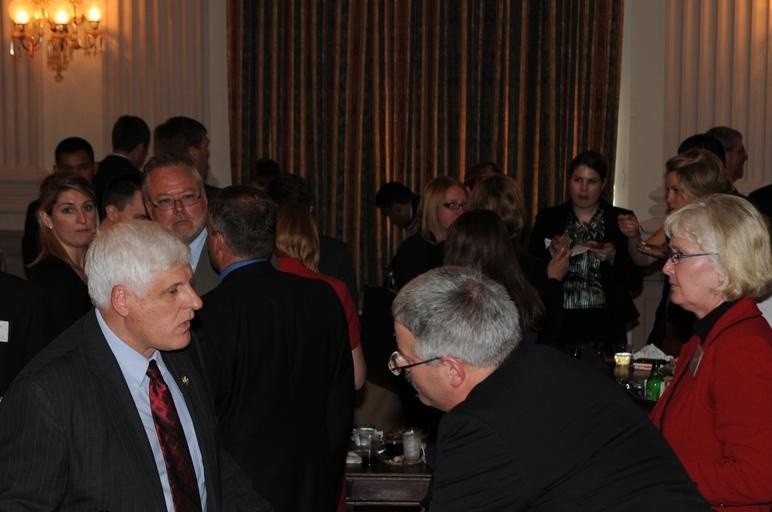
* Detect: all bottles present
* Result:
[646,361,660,401]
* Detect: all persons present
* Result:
[645,128,771,358]
[0,117,367,510]
[527,151,654,379]
[360,163,544,394]
[647,196,771,510]
[388,265,709,510]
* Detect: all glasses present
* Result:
[146,188,203,211]
[387,350,442,377]
[438,200,469,212]
[670,251,719,264]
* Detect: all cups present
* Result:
[614,352,631,365]
[350,424,423,471]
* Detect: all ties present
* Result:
[146,359,202,512]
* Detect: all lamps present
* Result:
[8,0,106,82]
